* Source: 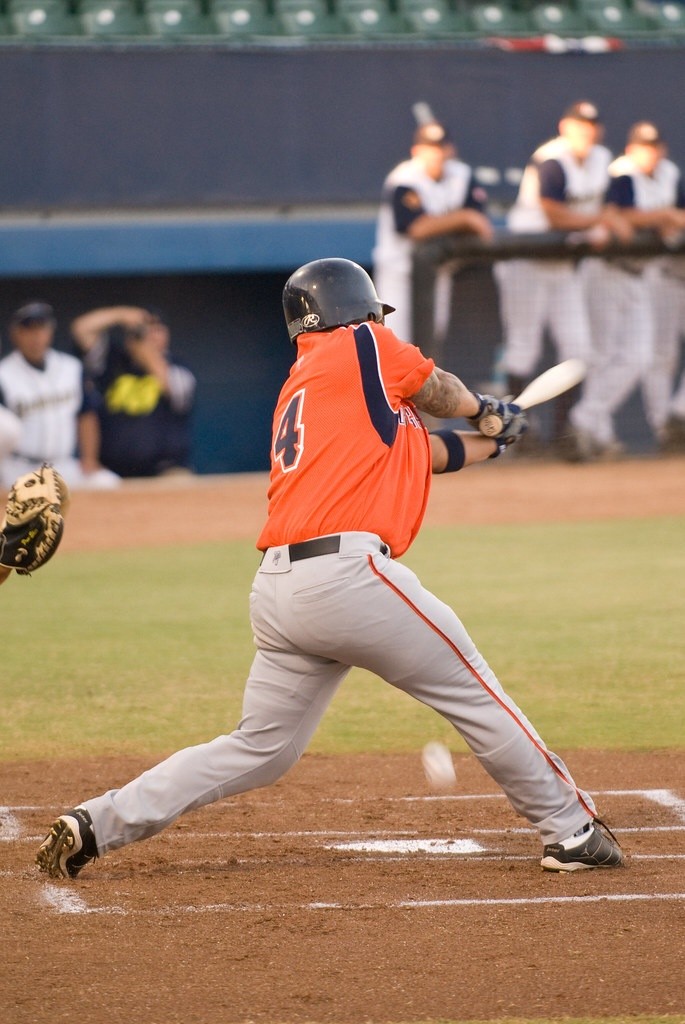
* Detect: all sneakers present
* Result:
[541,816,622,874]
[34,805,98,881]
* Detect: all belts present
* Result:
[288,535,386,561]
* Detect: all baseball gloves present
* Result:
[0,463,73,576]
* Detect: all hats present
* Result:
[559,103,601,134]
[623,122,663,149]
[411,125,449,149]
[12,294,55,321]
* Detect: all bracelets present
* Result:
[428,430,465,474]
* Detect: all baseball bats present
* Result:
[478,354,592,441]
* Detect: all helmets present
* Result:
[282,258,396,344]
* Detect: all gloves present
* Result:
[465,392,527,458]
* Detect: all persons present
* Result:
[33,256,630,879]
[370,102,685,462]
[0,302,196,584]
